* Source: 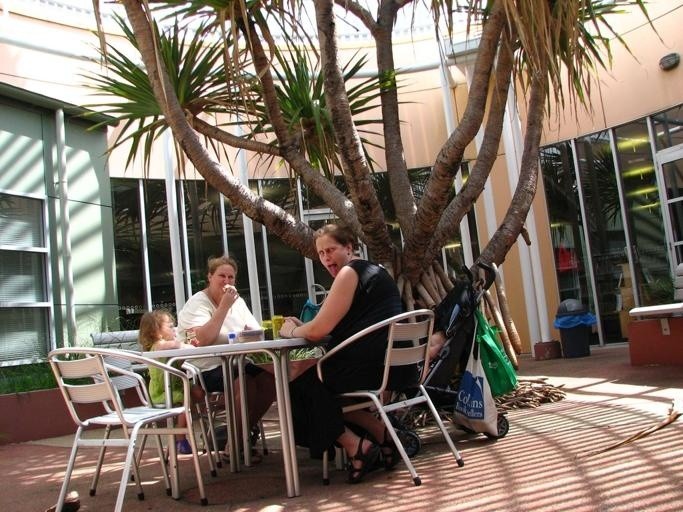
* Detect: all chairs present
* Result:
[320,306,468,489]
[46,331,267,511]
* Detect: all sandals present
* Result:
[346,437,375,482]
[377,424,403,470]
[175,439,193,455]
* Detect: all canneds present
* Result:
[273,315,284,340]
[262,320,273,329]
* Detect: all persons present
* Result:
[275,222,408,487]
[178,255,277,465]
[418,328,447,383]
[140,307,207,458]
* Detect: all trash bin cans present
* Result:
[554,299,591,358]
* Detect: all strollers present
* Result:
[396,260,510,459]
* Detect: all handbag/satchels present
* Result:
[452,308,518,438]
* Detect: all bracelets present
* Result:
[291,326,297,338]
